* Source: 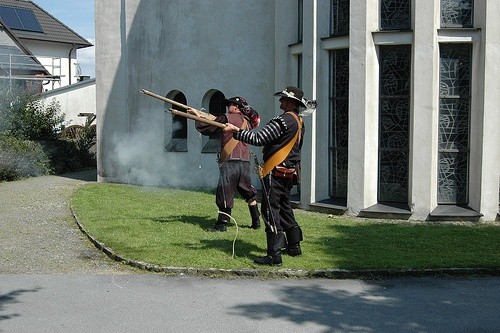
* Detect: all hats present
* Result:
[222,96,260,126]
[273,86,307,109]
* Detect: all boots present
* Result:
[254,231,284,266]
[248,204,261,229]
[281,226,301,257]
[206,207,231,232]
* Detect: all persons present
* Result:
[188,96,261,231]
[223,87,306,266]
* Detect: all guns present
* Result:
[164,107,228,129]
[137,88,218,121]
